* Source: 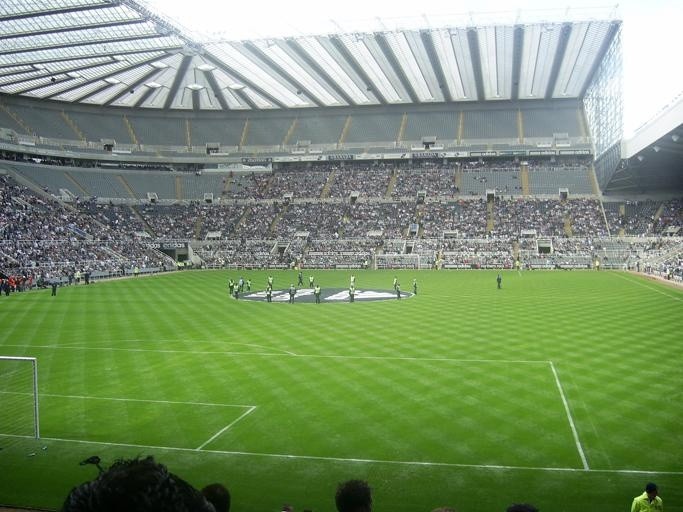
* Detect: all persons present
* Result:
[411,278,417,296]
[308,275,313,288]
[0,151,174,296]
[175,156,637,272]
[313,285,321,304]
[267,274,273,290]
[331,477,374,511]
[349,285,354,302]
[265,283,271,302]
[58,452,217,511]
[198,480,229,511]
[227,276,251,300]
[288,284,296,304]
[396,284,401,300]
[349,275,355,291]
[496,273,501,289]
[628,481,663,512]
[296,272,304,286]
[392,275,397,290]
[637,200,683,282]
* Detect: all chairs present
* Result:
[0,162,232,201]
[461,170,595,195]
